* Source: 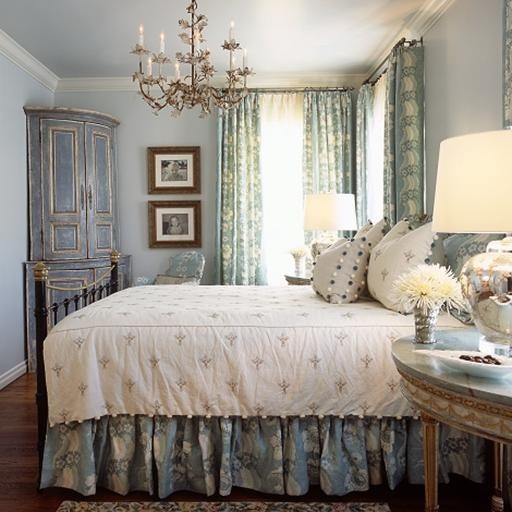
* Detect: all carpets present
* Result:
[55,501,391,512]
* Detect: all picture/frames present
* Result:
[149,200,202,248]
[147,146,202,194]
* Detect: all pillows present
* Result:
[152,274,197,285]
[353,218,391,261]
[368,217,436,314]
[310,238,370,304]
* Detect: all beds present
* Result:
[35,232,508,501]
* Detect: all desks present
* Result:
[390,326,512,512]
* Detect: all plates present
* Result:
[412,344,512,380]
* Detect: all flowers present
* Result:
[390,262,470,315]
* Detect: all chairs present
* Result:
[134,251,205,286]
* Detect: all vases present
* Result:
[413,302,438,345]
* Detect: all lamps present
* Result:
[431,129,512,355]
[304,193,357,243]
[129,0,255,119]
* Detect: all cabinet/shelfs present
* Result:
[23,105,119,260]
[24,254,131,372]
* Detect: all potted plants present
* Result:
[290,248,309,278]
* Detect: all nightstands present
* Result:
[284,274,312,285]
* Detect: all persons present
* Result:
[163,163,184,181]
[166,215,183,234]
